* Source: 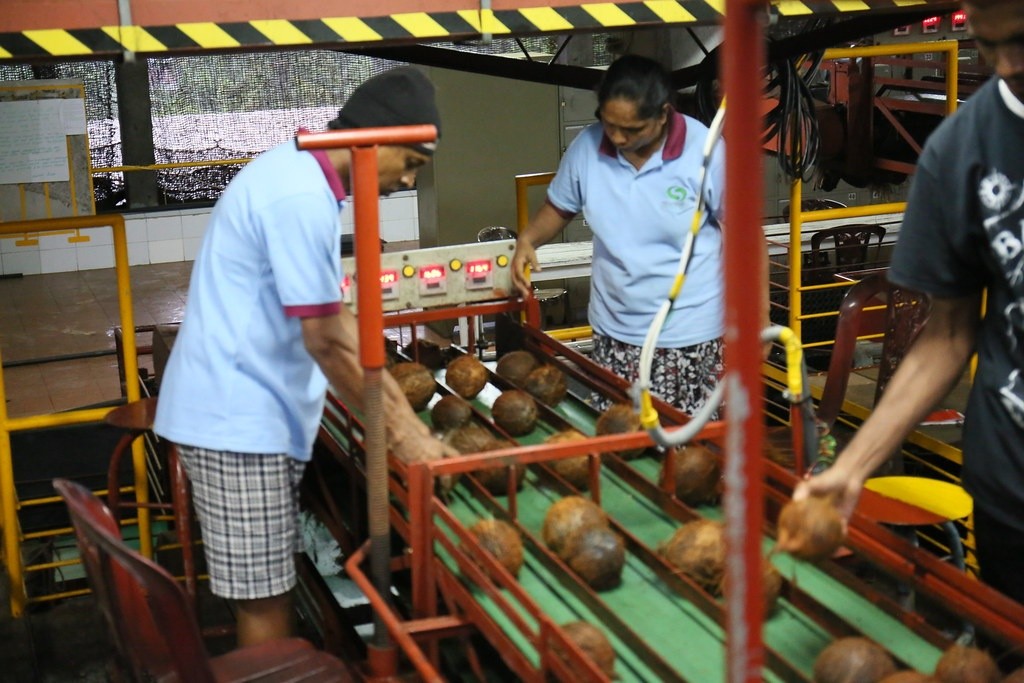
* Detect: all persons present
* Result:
[510,53,772,455]
[150,67,461,649]
[793,0,1024,679]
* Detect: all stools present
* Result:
[853,476,978,651]
[105,397,199,606]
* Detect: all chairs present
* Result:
[51,477,354,683]
[763,272,934,487]
[783,197,885,350]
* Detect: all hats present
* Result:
[338,66,441,156]
